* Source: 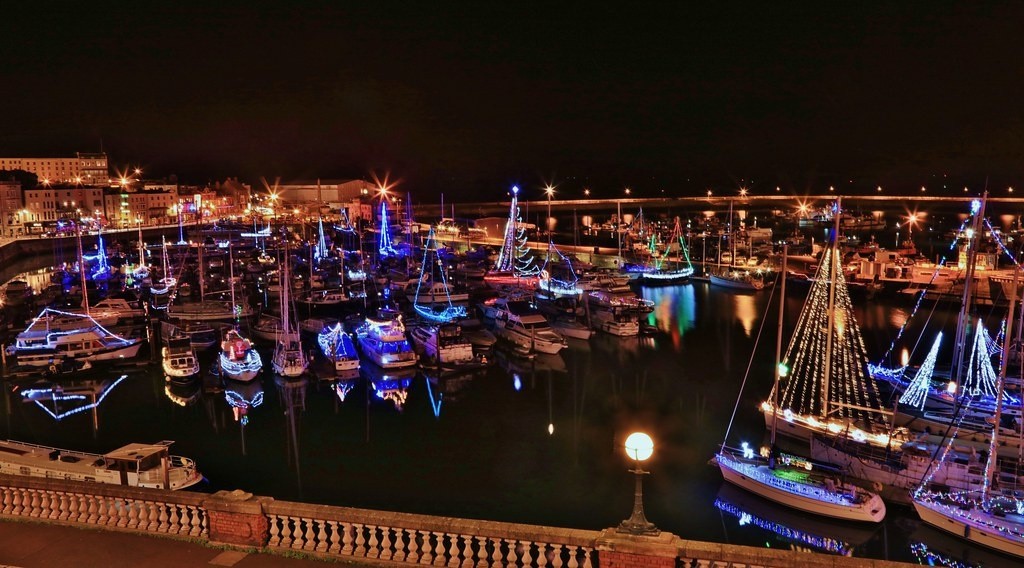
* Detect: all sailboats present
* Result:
[706,198,777,293]
[4,190,697,481]
[706,244,888,529]
[710,482,881,558]
[759,175,1024,562]
